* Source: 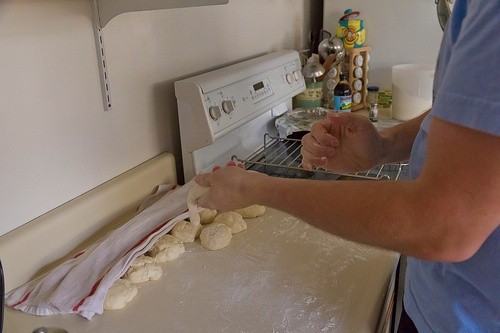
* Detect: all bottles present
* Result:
[334,74,352,113]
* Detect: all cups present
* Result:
[367,85,379,104]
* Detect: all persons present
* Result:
[192,1,500,333]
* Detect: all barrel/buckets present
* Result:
[391,64,435,122]
[336,20,367,48]
